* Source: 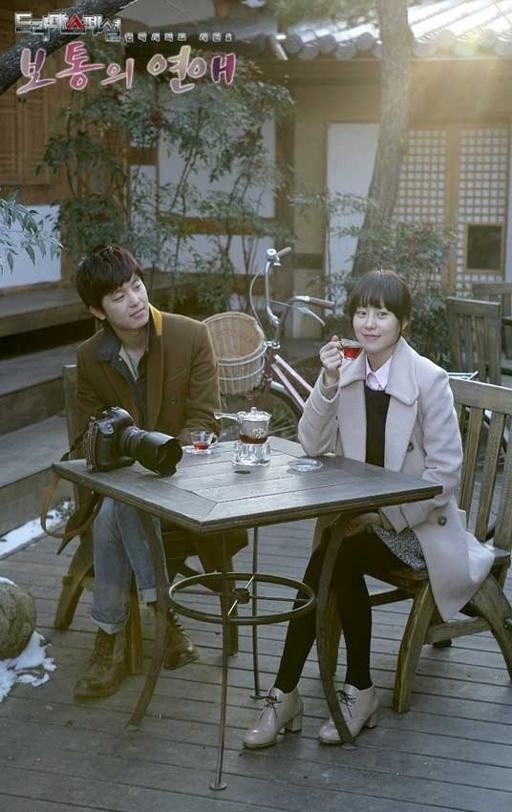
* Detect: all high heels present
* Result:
[243,686,304,750]
[319,685,379,746]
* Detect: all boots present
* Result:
[75,627,127,696]
[153,605,198,670]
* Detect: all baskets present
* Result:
[202,312,264,396]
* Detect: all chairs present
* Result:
[363,378,512,716]
[51,359,248,631]
[444,280,511,385]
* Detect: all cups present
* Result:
[193,432,215,451]
[213,407,272,443]
[337,338,362,360]
[231,443,273,467]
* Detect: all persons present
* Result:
[242,268,496,748]
[73,244,248,700]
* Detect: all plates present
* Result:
[183,447,217,455]
[288,458,323,472]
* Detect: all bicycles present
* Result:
[221,246,511,543]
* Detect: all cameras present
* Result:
[85,407,182,477]
